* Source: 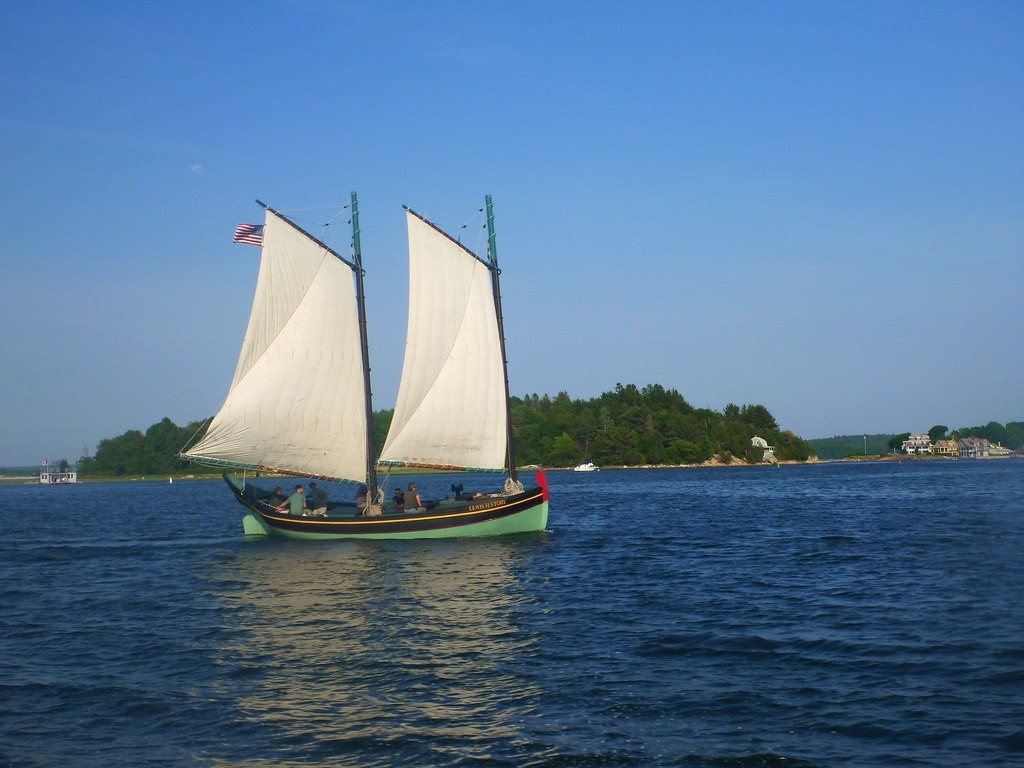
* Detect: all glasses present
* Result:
[395,492,400,494]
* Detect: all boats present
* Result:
[573,463,600,472]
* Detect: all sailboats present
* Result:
[173,190,551,541]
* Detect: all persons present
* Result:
[269,486,290,510]
[392,488,405,513]
[275,485,313,517]
[355,483,368,508]
[403,483,426,514]
[306,482,328,515]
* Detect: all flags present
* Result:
[233,223,265,248]
